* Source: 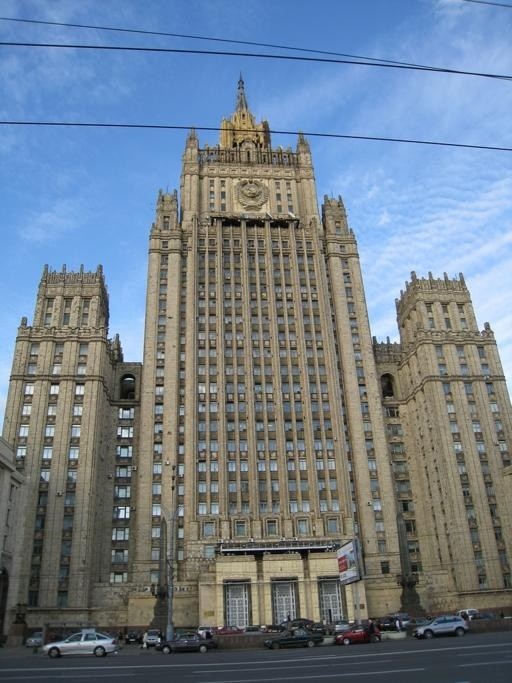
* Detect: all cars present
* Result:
[126,628,162,646]
[155,634,218,654]
[326,609,500,646]
[25,631,43,647]
[197,618,330,649]
[42,633,121,658]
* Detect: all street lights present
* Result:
[166,465,176,641]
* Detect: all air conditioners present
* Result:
[131,466,137,471]
[56,491,63,496]
[130,506,136,511]
